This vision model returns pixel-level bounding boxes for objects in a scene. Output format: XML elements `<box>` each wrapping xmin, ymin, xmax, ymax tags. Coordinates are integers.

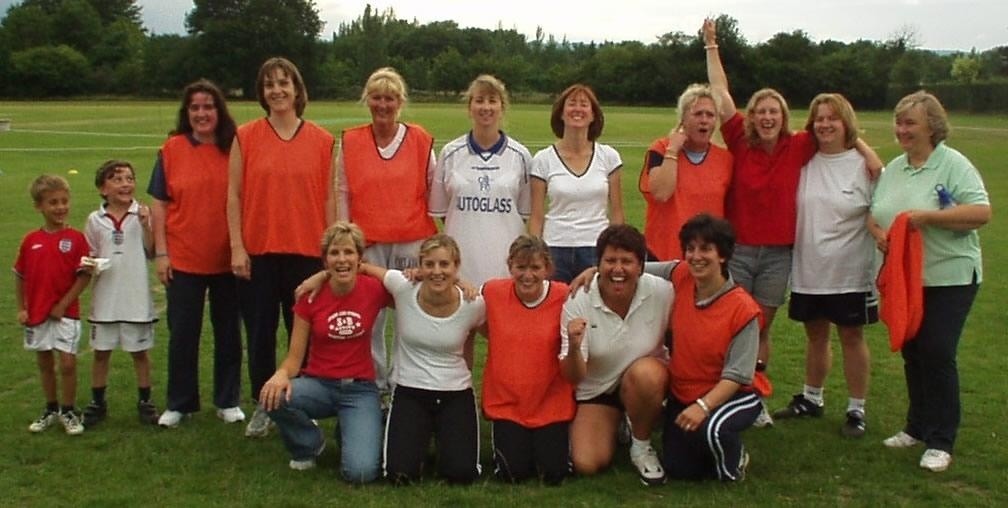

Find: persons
<box><xmin>637</xmin><ymin>85</ymin><xmax>734</xmax><ymax>265</ymax></box>
<box><xmin>13</xmin><ymin>174</ymin><xmax>90</xmax><ymax>441</ymax></box>
<box><xmin>557</xmin><ymin>222</ymin><xmax>677</xmax><ymax>486</ymax></box>
<box><xmin>865</xmin><ymin>90</ymin><xmax>992</xmax><ymax>469</ymax></box>
<box><xmin>565</xmin><ymin>211</ymin><xmax>766</xmax><ymax>488</ymax></box>
<box><xmin>770</xmin><ymin>92</ymin><xmax>887</xmax><ymax>439</ymax></box>
<box><xmin>291</xmin><ymin>233</ymin><xmax>488</xmax><ymax>480</ymax></box>
<box><xmin>144</xmin><ymin>79</ymin><xmax>249</xmax><ymax>429</ymax></box>
<box><xmin>224</xmin><ymin>56</ymin><xmax>337</xmax><ymax>438</ymax></box>
<box><xmin>529</xmin><ymin>83</ymin><xmax>625</xmax><ymax>279</ymax></box>
<box><xmin>323</xmin><ymin>67</ymin><xmax>441</xmax><ymax>419</ymax></box>
<box><xmin>82</xmin><ymin>158</ymin><xmax>157</xmax><ymax>429</ymax></box>
<box><xmin>703</xmin><ymin>15</ymin><xmax>880</xmax><ymax>434</ymax></box>
<box><xmin>398</xmin><ymin>233</ymin><xmax>574</xmax><ymax>485</ymax></box>
<box><xmin>427</xmin><ymin>75</ymin><xmax>538</xmax><ymax>378</ymax></box>
<box><xmin>258</xmin><ymin>221</ymin><xmax>396</xmax><ymax>487</ymax></box>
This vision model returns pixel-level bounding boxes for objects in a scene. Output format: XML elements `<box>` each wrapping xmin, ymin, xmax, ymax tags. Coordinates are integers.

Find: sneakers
<box><xmin>157</xmin><ymin>408</ymin><xmax>192</xmax><ymax>428</ymax></box>
<box><xmin>217</xmin><ymin>406</ymin><xmax>245</xmax><ymax>424</ymax></box>
<box><xmin>29</xmin><ymin>409</ymin><xmax>62</xmax><ymax>433</ymax></box>
<box><xmin>136</xmin><ymin>399</ymin><xmax>159</xmax><ymax>425</ymax></box>
<box><xmin>289</xmin><ymin>440</ymin><xmax>326</xmax><ymax>471</ymax></box>
<box><xmin>773</xmin><ymin>393</ymin><xmax>824</xmax><ymax>419</ymax></box>
<box><xmin>842</xmin><ymin>410</ymin><xmax>865</xmax><ymax>438</ymax></box>
<box><xmin>883</xmin><ymin>430</ymin><xmax>921</xmax><ymax>447</ymax></box>
<box><xmin>62</xmin><ymin>400</ymin><xmax>108</xmax><ymax>435</ymax></box>
<box><xmin>629</xmin><ymin>446</ymin><xmax>666</xmax><ymax>486</ymax></box>
<box><xmin>245</xmin><ymin>403</ymin><xmax>275</xmax><ymax>439</ymax></box>
<box><xmin>919</xmin><ymin>448</ymin><xmax>951</xmax><ymax>473</ymax></box>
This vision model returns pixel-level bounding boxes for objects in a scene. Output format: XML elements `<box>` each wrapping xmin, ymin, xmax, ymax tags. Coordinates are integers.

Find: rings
<box><xmin>685</xmin><ymin>424</ymin><xmax>690</xmax><ymax>430</ymax></box>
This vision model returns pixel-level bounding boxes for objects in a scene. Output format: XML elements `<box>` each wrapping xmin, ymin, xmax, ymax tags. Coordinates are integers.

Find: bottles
<box><xmin>935</xmin><ymin>181</ymin><xmax>973</xmax><ymax>240</ymax></box>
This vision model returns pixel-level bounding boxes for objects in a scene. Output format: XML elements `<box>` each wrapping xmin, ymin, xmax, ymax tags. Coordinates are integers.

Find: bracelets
<box><xmin>694</xmin><ymin>397</ymin><xmax>712</xmax><ymax>419</ymax></box>
<box><xmin>152</xmin><ymin>249</ymin><xmax>170</xmax><ymax>261</ymax></box>
<box><xmin>323</xmin><ymin>268</ymin><xmax>331</xmax><ymax>281</ymax></box>
<box><xmin>662</xmin><ymin>150</ymin><xmax>678</xmax><ymax>164</ymax></box>
<box><xmin>703</xmin><ymin>40</ymin><xmax>720</xmax><ymax>49</ymax></box>
